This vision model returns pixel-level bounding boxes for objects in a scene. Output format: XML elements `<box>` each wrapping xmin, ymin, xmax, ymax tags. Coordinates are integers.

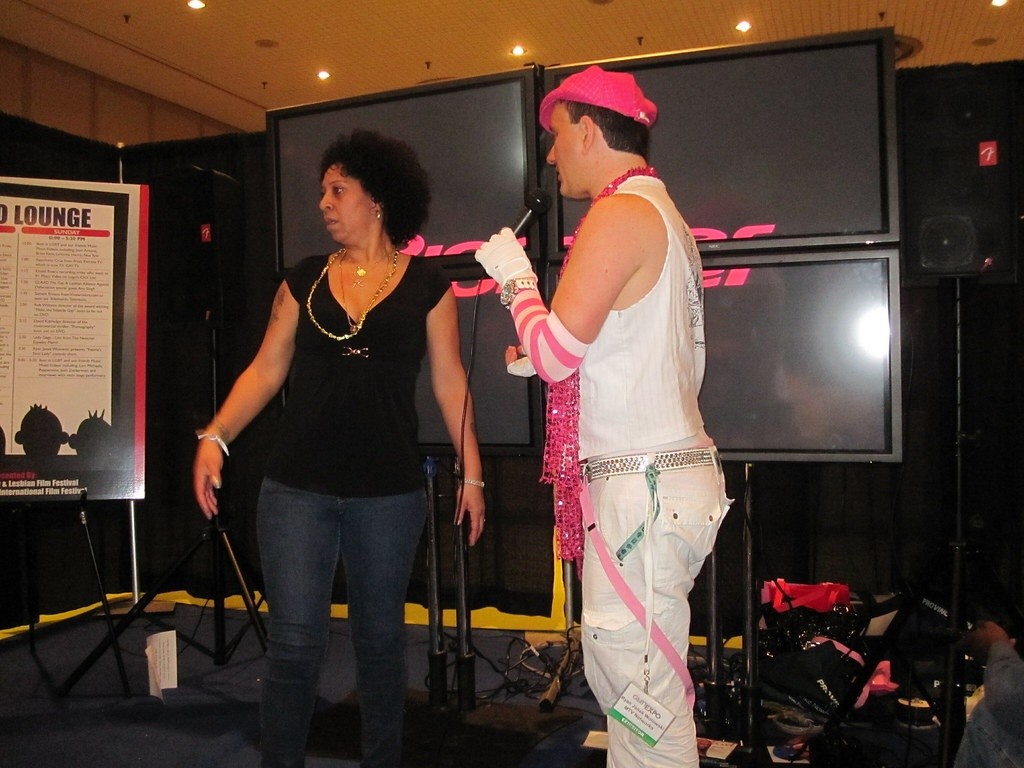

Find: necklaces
<box><xmin>307</xmin><ymin>245</ymin><xmax>399</xmax><ymax>341</ymax></box>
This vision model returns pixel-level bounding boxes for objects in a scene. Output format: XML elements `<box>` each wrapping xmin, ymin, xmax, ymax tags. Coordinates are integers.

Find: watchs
<box><xmin>500</xmin><ymin>278</ymin><xmax>537</xmax><ymax>306</ymax></box>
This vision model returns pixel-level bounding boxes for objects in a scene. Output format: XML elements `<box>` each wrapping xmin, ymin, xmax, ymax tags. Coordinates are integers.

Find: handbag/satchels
<box><xmin>751</xmin><ymin>577</ymin><xmax>961</xmax><ymax>752</ymax></box>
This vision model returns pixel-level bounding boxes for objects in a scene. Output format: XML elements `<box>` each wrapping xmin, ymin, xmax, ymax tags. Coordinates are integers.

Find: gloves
<box><xmin>475</xmin><ymin>225</ymin><xmax>538</xmax><ymax>286</ymax></box>
<box><xmin>506</xmin><ymin>345</ymin><xmax>537</xmax><ymax>377</ymax></box>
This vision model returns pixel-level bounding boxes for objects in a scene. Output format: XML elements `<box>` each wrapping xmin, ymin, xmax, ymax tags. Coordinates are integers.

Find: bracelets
<box><xmin>195</xmin><ymin>419</ymin><xmax>229</xmax><ymax>456</ymax></box>
<box><xmin>458</xmin><ymin>478</ymin><xmax>484</xmax><ymax>488</ymax></box>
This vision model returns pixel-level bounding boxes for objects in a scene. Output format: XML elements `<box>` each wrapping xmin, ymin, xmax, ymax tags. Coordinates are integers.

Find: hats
<box><xmin>539</xmin><ymin>64</ymin><xmax>657</xmax><ymax>134</ymax></box>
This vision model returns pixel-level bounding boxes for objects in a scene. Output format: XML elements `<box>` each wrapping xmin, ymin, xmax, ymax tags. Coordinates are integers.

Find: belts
<box><xmin>580</xmin><ymin>449</ymin><xmax>720</xmax><ymax>486</ymax></box>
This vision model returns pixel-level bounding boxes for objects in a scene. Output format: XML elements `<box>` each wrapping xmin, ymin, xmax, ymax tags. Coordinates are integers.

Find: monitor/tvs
<box><xmin>263</xmin><ymin>29</ymin><xmax>903</xmax><ymax>465</ymax></box>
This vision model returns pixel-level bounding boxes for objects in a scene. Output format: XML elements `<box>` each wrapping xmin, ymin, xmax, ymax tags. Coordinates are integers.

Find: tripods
<box><xmin>52</xmin><ymin>323</ymin><xmax>273</xmax><ymax>698</ymax></box>
<box><xmin>819</xmin><ymin>277</ymin><xmax>1024</xmax><ymax>768</ymax></box>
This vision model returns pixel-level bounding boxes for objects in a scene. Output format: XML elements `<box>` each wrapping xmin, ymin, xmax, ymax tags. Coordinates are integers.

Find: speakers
<box><xmin>149</xmin><ymin>136</ymin><xmax>247</xmax><ymax>331</ymax></box>
<box><xmin>894</xmin><ymin>62</ymin><xmax>1024</xmax><ymax>287</ymax></box>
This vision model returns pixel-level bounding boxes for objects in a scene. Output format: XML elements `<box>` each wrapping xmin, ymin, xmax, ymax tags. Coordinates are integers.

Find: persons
<box><xmin>952</xmin><ymin>620</ymin><xmax>1024</xmax><ymax>768</ymax></box>
<box><xmin>475</xmin><ymin>65</ymin><xmax>735</xmax><ymax>768</ymax></box>
<box><xmin>194</xmin><ymin>128</ymin><xmax>485</xmax><ymax>768</ymax></box>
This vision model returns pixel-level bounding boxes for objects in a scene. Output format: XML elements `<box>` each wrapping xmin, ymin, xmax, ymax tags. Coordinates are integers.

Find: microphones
<box><xmin>512</xmin><ymin>188</ymin><xmax>549</xmax><ymax>239</ymax></box>
